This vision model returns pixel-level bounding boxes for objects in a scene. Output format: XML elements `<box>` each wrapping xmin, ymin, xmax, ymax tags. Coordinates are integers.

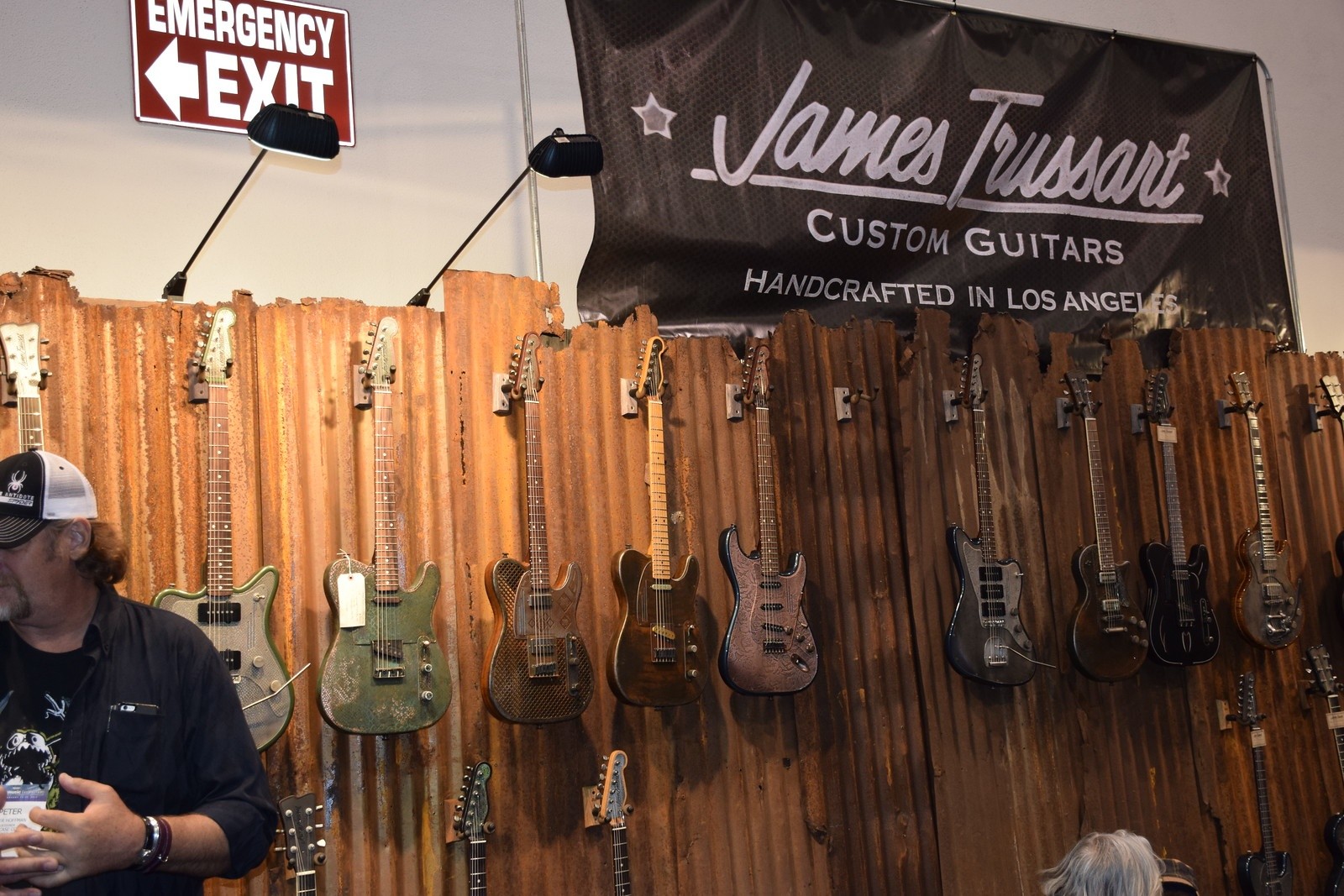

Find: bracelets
<box><xmin>142</xmin><ymin>816</ymin><xmax>172</xmax><ymax>874</ymax></box>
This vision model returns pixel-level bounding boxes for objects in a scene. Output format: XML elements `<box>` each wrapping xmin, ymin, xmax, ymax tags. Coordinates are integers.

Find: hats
<box><xmin>0</xmin><ymin>445</ymin><xmax>97</xmax><ymax>549</ymax></box>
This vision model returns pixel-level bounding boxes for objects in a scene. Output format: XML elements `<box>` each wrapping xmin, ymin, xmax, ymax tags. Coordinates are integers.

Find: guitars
<box><xmin>942</xmin><ymin>352</ymin><xmax>1057</xmax><ymax>687</ymax></box>
<box><xmin>1139</xmin><ymin>370</ymin><xmax>1221</xmax><ymax>667</ymax></box>
<box><xmin>1059</xmin><ymin>367</ymin><xmax>1150</xmax><ymax>685</ymax></box>
<box><xmin>0</xmin><ymin>320</ymin><xmax>54</xmax><ymax>453</ymax></box>
<box><xmin>1302</xmin><ymin>644</ymin><xmax>1344</xmax><ymax>896</ymax></box>
<box><xmin>717</xmin><ymin>343</ymin><xmax>822</xmax><ymax>698</ymax></box>
<box><xmin>273</xmin><ymin>791</ymin><xmax>327</xmax><ymax>896</ymax></box>
<box><xmin>1315</xmin><ymin>374</ymin><xmax>1343</xmax><ymax>575</ymax></box>
<box><xmin>607</xmin><ymin>335</ymin><xmax>710</xmax><ymax>708</ymax></box>
<box><xmin>452</xmin><ymin>761</ymin><xmax>497</xmax><ymax>895</ymax></box>
<box><xmin>479</xmin><ymin>331</ymin><xmax>595</xmax><ymax>726</ymax></box>
<box><xmin>314</xmin><ymin>315</ymin><xmax>454</xmax><ymax>737</ymax></box>
<box><xmin>149</xmin><ymin>307</ymin><xmax>296</xmax><ymax>753</ymax></box>
<box><xmin>591</xmin><ymin>749</ymin><xmax>635</xmax><ymax>896</ymax></box>
<box><xmin>1235</xmin><ymin>671</ymin><xmax>1294</xmax><ymax>896</ymax></box>
<box><xmin>1224</xmin><ymin>370</ymin><xmax>1306</xmax><ymax>652</ymax></box>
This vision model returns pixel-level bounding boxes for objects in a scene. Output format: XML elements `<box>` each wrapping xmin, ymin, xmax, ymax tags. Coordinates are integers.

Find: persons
<box><xmin>1038</xmin><ymin>830</ymin><xmax>1164</xmax><ymax>896</ymax></box>
<box><xmin>0</xmin><ymin>447</ymin><xmax>277</xmax><ymax>896</ymax></box>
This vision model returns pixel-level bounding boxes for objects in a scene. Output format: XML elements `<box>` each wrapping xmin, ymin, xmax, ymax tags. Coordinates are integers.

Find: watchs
<box><xmin>137</xmin><ymin>811</ymin><xmax>155</xmax><ymax>866</ymax></box>
